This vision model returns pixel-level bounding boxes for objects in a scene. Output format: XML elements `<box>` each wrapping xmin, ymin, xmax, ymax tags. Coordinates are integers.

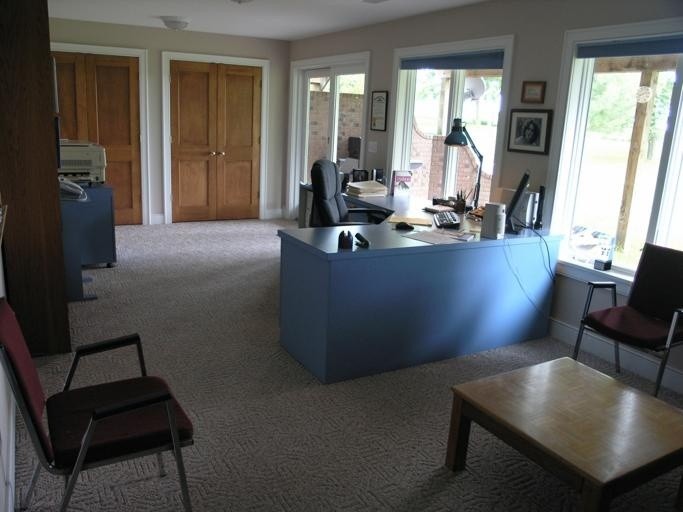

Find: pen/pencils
<box><xmin>456</xmin><ymin>189</ymin><xmax>473</xmax><ymax>200</ymax></box>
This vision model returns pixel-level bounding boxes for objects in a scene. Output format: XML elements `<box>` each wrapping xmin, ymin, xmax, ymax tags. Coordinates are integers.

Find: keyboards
<box><xmin>433</xmin><ymin>212</ymin><xmax>460</xmax><ymax>228</ymax></box>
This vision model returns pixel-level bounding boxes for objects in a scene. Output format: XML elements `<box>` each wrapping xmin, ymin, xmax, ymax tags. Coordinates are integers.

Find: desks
<box><xmin>277</xmin><ymin>185</ymin><xmax>561</xmax><ymax>382</ymax></box>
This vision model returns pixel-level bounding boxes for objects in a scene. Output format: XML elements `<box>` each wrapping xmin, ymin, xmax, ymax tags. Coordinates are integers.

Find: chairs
<box><xmin>308</xmin><ymin>160</ymin><xmax>387</xmax><ymax>227</ymax></box>
<box><xmin>573</xmin><ymin>242</ymin><xmax>683</xmax><ymax>398</ymax></box>
<box><xmin>1</xmin><ymin>296</ymin><xmax>194</xmax><ymax>512</ymax></box>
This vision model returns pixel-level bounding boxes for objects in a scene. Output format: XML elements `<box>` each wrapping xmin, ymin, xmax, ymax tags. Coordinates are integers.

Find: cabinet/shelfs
<box><xmin>0</xmin><ymin>0</ymin><xmax>72</xmax><ymax>358</ymax></box>
<box><xmin>61</xmin><ymin>185</ymin><xmax>117</xmax><ymax>301</ymax></box>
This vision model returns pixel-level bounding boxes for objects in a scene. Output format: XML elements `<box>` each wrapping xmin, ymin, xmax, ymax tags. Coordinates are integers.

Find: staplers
<box><xmin>355</xmin><ymin>233</ymin><xmax>369</xmax><ymax>249</ymax></box>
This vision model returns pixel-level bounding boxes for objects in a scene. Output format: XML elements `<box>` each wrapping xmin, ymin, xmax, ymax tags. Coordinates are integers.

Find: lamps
<box><xmin>444</xmin><ymin>118</ymin><xmax>484</xmax><ymax>210</ymax></box>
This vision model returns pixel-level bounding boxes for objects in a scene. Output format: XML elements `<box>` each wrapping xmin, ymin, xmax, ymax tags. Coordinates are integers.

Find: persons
<box><xmin>516</xmin><ymin>119</ymin><xmax>540</xmax><ymax>146</ymax></box>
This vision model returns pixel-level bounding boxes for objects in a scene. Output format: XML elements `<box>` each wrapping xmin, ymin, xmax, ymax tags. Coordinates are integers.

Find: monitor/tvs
<box><xmin>505</xmin><ymin>171</ymin><xmax>529</xmax><ymax>234</ymax></box>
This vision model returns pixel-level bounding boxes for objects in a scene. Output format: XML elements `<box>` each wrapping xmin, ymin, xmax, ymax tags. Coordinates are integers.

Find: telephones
<box><xmin>61</xmin><ymin>180</ymin><xmax>83</xmax><ymax>198</ymax></box>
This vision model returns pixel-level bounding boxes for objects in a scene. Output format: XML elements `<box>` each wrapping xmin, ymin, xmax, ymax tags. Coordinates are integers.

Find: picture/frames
<box><xmin>521</xmin><ymin>81</ymin><xmax>546</xmax><ymax>103</ymax></box>
<box><xmin>507</xmin><ymin>109</ymin><xmax>553</xmax><ymax>155</ymax></box>
<box><xmin>370</xmin><ymin>91</ymin><xmax>388</xmax><ymax>131</ymax></box>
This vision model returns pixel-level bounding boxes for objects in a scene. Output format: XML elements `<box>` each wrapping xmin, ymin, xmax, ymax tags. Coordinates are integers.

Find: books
<box><xmin>346</xmin><ymin>180</ymin><xmax>387</xmax><ymax>197</ymax></box>
<box><xmin>452</xmin><ymin>234</ymin><xmax>474</xmax><ymax>241</ymax></box>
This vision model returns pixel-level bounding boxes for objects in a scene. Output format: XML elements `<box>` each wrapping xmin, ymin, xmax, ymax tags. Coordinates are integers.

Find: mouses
<box><xmin>396</xmin><ymin>222</ymin><xmax>414</xmax><ymax>229</ymax></box>
